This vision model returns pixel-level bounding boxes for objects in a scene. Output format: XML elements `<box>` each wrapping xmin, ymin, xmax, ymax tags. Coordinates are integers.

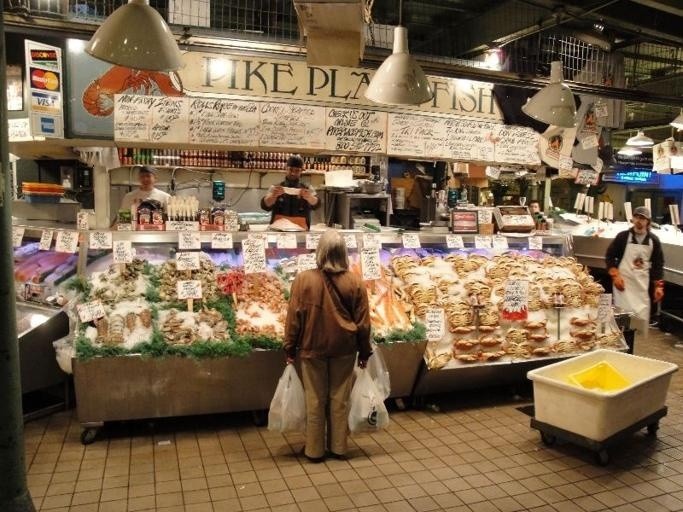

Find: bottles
<box><xmin>119</xmin><ymin>147</ymin><xmax>366</xmax><ymax>174</ymax></box>
<box><xmin>77</xmin><ymin>208</ymin><xmax>97</xmax><ymax>229</ymax></box>
<box><xmin>533</xmin><ymin>212</ymin><xmax>554</xmax><ymax>230</ymax></box>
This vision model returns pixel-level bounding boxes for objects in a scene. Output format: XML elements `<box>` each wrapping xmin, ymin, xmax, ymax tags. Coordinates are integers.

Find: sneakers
<box><xmin>301</xmin><ymin>445</ymin><xmax>345</xmax><ymax>462</ymax></box>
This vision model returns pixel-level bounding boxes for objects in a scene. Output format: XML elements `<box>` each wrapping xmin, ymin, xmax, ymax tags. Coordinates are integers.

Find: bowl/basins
<box><xmin>357</xmin><ymin>180</ymin><xmax>384</xmax><ymax>194</ymax></box>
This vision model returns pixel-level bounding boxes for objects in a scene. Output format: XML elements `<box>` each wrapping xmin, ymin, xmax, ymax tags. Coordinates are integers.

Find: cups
<box><xmin>520</xmin><ymin>197</ymin><xmax>527</xmax><ymax>206</ymax></box>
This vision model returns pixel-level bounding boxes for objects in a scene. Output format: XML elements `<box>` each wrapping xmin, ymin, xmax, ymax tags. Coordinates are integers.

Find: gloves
<box><xmin>607</xmin><ymin>267</ymin><xmax>625</xmax><ymax>292</ymax></box>
<box><xmin>653</xmin><ymin>280</ymin><xmax>664</xmax><ymax>302</ymax></box>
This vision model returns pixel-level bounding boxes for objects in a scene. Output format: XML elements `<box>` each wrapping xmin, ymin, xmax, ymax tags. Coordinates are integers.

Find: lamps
<box><xmin>616</xmin><ymin>106</ymin><xmax>683</xmax><ymax>156</ymax></box>
<box><xmin>364</xmin><ymin>2</ymin><xmax>435</xmax><ymax>105</ymax></box>
<box><xmin>521</xmin><ymin>1</ymin><xmax>576</xmax><ymax>130</ymax></box>
<box><xmin>83</xmin><ymin>2</ymin><xmax>184</xmax><ymax>73</ymax></box>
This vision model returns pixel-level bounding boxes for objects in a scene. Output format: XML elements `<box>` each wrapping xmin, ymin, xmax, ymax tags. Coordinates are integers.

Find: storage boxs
<box><xmin>526</xmin><ymin>343</ymin><xmax>681</xmax><ymax>441</ymax></box>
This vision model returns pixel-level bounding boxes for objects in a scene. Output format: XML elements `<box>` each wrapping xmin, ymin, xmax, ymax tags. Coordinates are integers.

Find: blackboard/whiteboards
<box><xmin>388</xmin><ymin>111</ymin><xmax>446</xmax><ymax>159</ymax></box>
<box><xmin>187</xmin><ymin>95</ymin><xmax>260</xmax><ymax>151</ymax></box>
<box><xmin>326</xmin><ymin>103</ymin><xmax>388</xmax><ymax>156</ymax></box>
<box><xmin>495</xmin><ymin>115</ymin><xmax>540</xmax><ymax>169</ymax></box>
<box><xmin>113</xmin><ymin>95</ymin><xmax>189</xmax><ymax>148</ymax></box>
<box><xmin>446</xmin><ymin>113</ymin><xmax>494</xmax><ymax>165</ymax></box>
<box><xmin>260</xmin><ymin>101</ymin><xmax>326</xmax><ymax>152</ymax></box>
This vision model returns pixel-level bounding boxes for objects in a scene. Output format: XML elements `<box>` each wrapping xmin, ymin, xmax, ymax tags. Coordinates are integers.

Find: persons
<box><xmin>283</xmin><ymin>229</ymin><xmax>373</xmax><ymax>463</ymax></box>
<box><xmin>112</xmin><ymin>164</ymin><xmax>171</xmax><ymax>230</ymax></box>
<box><xmin>602</xmin><ymin>206</ymin><xmax>665</xmax><ymax>354</ymax></box>
<box><xmin>260</xmin><ymin>155</ymin><xmax>321</xmax><ymax>231</ymax></box>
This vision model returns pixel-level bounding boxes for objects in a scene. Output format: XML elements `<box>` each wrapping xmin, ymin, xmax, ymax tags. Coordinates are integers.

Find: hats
<box><xmin>287</xmin><ymin>156</ymin><xmax>303</xmax><ymax>168</ymax></box>
<box><xmin>139</xmin><ymin>167</ymin><xmax>156</xmax><ymax>173</ymax></box>
<box><xmin>633</xmin><ymin>206</ymin><xmax>650</xmax><ymax>219</ymax></box>
